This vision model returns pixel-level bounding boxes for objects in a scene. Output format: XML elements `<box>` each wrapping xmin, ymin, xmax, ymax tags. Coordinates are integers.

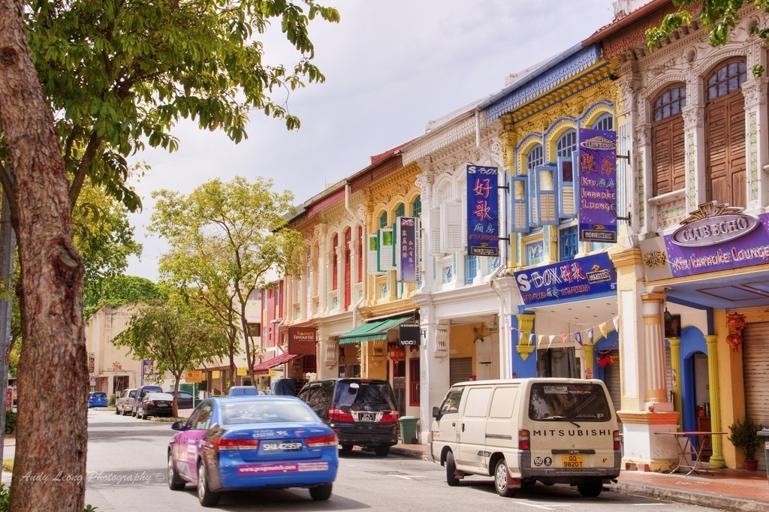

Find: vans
<box><xmin>298</xmin><ymin>378</ymin><xmax>401</xmax><ymax>459</ymax></box>
<box><xmin>430</xmin><ymin>376</ymin><xmax>623</xmax><ymax>497</ymax></box>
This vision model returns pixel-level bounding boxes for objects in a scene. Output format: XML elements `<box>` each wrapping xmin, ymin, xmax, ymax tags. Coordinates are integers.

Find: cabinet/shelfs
<box><xmin>699</xmin><ymin>415</ymin><xmax>713</xmax><ymax>456</ymax></box>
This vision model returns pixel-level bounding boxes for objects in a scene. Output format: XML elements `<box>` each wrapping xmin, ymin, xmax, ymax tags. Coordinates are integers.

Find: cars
<box><xmin>168</xmin><ymin>386</ymin><xmax>338</xmax><ymax>508</ymax></box>
<box><xmin>115</xmin><ymin>385</ymin><xmax>203</xmax><ymax>419</ymax></box>
<box><xmin>87</xmin><ymin>391</ymin><xmax>108</xmax><ymax>408</ymax></box>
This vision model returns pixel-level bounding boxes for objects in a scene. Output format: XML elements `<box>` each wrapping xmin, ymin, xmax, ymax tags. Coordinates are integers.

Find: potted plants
<box><xmin>727</xmin><ymin>417</ymin><xmax>763</xmax><ymax>470</ymax></box>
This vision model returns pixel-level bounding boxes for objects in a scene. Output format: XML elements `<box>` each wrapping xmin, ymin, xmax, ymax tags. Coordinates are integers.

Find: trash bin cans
<box><xmin>398</xmin><ymin>415</ymin><xmax>420</xmax><ymax>444</ymax></box>
<box><xmin>757</xmin><ymin>428</ymin><xmax>769</xmax><ymax>480</ymax></box>
<box><xmin>195</xmin><ymin>400</ymin><xmax>201</xmax><ymax>407</ymax></box>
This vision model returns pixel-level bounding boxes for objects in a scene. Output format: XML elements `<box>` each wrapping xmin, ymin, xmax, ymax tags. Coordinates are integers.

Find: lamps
<box><xmin>387</xmin><ymin>343</ymin><xmax>404</xmax><ymax>364</ymax></box>
<box><xmin>725</xmin><ymin>312</ymin><xmax>746</xmax><ymax>333</ymax></box>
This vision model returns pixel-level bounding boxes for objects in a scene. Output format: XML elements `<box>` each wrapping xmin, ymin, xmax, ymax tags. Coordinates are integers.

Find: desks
<box><xmin>654</xmin><ymin>430</ymin><xmax>728</xmax><ymax>476</ymax></box>
